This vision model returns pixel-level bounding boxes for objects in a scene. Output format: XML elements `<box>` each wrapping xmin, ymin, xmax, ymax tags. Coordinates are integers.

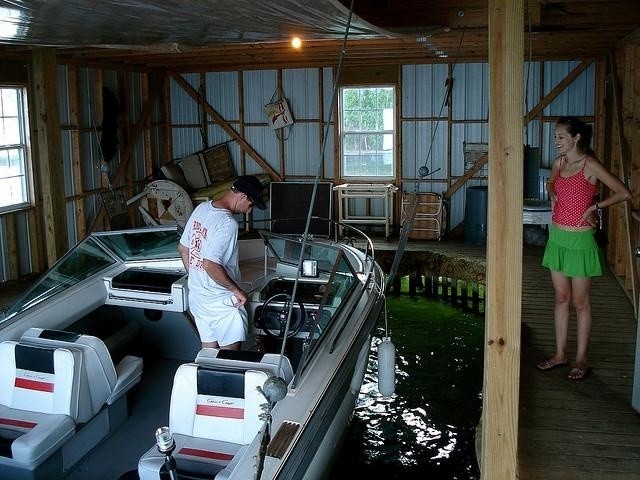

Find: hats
<box><xmin>231</xmin><ymin>175</ymin><xmax>267</xmax><ymax>210</ymax></box>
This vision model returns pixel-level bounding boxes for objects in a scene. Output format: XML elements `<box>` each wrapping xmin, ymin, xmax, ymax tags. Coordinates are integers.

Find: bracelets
<box><xmin>595</xmin><ymin>202</ymin><xmax>605</xmax><ymax>211</ymax></box>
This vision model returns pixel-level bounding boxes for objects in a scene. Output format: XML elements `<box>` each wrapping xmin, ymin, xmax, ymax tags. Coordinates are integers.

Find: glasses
<box><xmin>248</xmin><ymin>196</ymin><xmax>256</xmax><ymax>207</ymax></box>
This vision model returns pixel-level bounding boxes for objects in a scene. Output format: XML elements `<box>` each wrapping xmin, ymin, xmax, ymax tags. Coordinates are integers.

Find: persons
<box><xmin>536</xmin><ymin>118</ymin><xmax>632</xmax><ymax>380</ymax></box>
<box><xmin>177</xmin><ymin>175</ymin><xmax>267</xmax><ymax>351</ymax></box>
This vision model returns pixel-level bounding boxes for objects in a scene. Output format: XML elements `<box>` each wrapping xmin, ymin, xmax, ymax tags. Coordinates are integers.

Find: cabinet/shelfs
<box><xmin>332</xmin><ymin>183</ymin><xmax>392</xmax><ymax>243</ymax></box>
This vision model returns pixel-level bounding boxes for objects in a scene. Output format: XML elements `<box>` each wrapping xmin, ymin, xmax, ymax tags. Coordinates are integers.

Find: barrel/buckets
<box><xmin>465</xmin><ymin>186</ymin><xmax>486</xmax><ymax>242</ymax></box>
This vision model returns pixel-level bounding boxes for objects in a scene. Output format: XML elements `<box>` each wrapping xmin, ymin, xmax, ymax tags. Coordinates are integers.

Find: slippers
<box><xmin>536</xmin><ymin>358</ymin><xmax>568</xmax><ymax>371</ymax></box>
<box><xmin>567</xmin><ymin>367</ymin><xmax>590</xmax><ymax>381</ymax></box>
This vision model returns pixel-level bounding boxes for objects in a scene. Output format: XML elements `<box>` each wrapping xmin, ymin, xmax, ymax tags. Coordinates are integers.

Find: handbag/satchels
<box><xmin>265</xmin><ymin>87</ymin><xmax>294</xmax><ymax>141</ymax></box>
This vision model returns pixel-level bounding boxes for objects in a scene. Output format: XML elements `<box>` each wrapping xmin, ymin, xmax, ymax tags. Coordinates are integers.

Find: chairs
<box><xmin>136</xmin><ymin>347</ymin><xmax>295</xmax><ymax>480</ymax></box>
<box><xmin>0</xmin><ymin>329</ymin><xmax>145</xmax><ymax>472</ymax></box>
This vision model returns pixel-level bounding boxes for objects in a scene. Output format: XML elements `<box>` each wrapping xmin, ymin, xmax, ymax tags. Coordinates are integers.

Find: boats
<box><xmin>0</xmin><ymin>214</ymin><xmax>389</xmax><ymax>480</ymax></box>
